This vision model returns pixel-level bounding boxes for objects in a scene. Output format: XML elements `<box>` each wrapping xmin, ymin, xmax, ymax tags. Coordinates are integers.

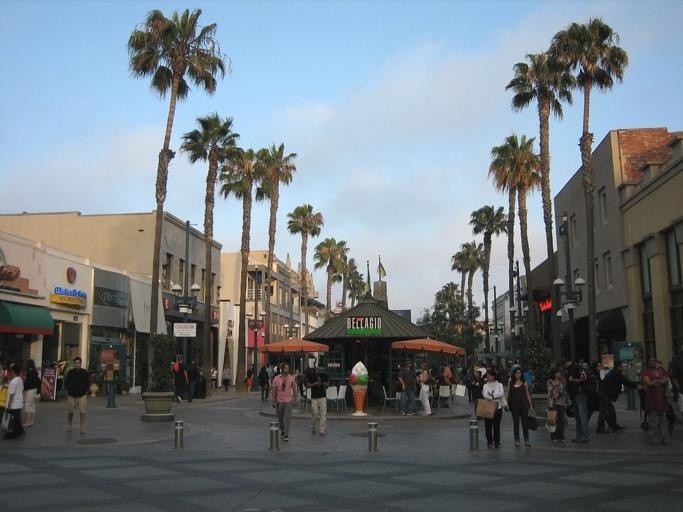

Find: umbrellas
<box><xmin>258</xmin><ymin>338</ymin><xmax>331</xmax><ymax>362</ymax></box>
<box><xmin>392</xmin><ymin>336</ymin><xmax>466</xmax><ymax>363</ymax></box>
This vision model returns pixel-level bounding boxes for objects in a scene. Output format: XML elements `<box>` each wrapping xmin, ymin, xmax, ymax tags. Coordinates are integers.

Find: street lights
<box><xmin>245</xmin><ymin>310</ymin><xmax>266</xmax><ymax>392</ymax></box>
<box><xmin>171</xmin><ymin>283</ymin><xmax>201</xmax><ymax>367</ymax></box>
<box><xmin>551</xmin><ymin>277</ymin><xmax>585</xmax><ymax>361</ymax></box>
<box><xmin>283</xmin><ymin>323</ymin><xmax>299</xmax><ymax>375</ymax></box>
<box><xmin>486</xmin><ymin>322</ymin><xmax>505</xmax><ymax>369</ymax></box>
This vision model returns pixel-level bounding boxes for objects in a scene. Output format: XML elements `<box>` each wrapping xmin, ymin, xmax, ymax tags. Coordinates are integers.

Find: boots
<box><xmin>80</xmin><ymin>413</ymin><xmax>87</xmax><ymax>434</ymax></box>
<box><xmin>67</xmin><ymin>413</ymin><xmax>73</xmax><ymax>432</ymax></box>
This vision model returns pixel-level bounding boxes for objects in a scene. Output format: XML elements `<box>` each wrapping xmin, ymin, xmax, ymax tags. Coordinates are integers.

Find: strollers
<box><xmin>637</xmin><ymin>388</ymin><xmax>682</xmax><ymax>434</ymax></box>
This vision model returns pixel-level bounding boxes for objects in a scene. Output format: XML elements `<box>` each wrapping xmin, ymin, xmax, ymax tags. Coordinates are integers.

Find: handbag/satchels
<box><xmin>1</xmin><ymin>413</ymin><xmax>14</xmax><ymax>433</ymax></box>
<box><xmin>565</xmin><ymin>381</ymin><xmax>581</xmax><ymax>394</ymax></box>
<box><xmin>665</xmin><ymin>377</ymin><xmax>674</xmax><ymax>398</ymax></box>
<box><xmin>476</xmin><ymin>398</ymin><xmax>576</xmax><ymax>433</ymax></box>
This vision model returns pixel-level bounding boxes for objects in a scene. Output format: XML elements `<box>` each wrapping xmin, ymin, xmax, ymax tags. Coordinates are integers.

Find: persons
<box><xmin>506</xmin><ymin>365</ymin><xmax>533</xmax><ymax>447</ymax></box>
<box><xmin>482</xmin><ymin>369</ymin><xmax>509</xmax><ymax>448</ymax></box>
<box><xmin>0</xmin><ymin>357</ymin><xmax>26</xmax><ymax>439</ymax></box>
<box><xmin>65</xmin><ymin>357</ymin><xmax>89</xmax><ymax>434</ymax></box>
<box><xmin>306</xmin><ymin>363</ymin><xmax>330</xmax><ymax>435</ymax></box>
<box><xmin>546</xmin><ymin>351</ymin><xmax>683</xmax><ymax>445</ymax></box>
<box><xmin>397</xmin><ymin>356</ymin><xmax>492</xmax><ymax>416</ymax></box>
<box><xmin>520</xmin><ymin>365</ymin><xmax>538</xmax><ymax>395</ymax></box>
<box><xmin>272</xmin><ymin>363</ymin><xmax>296</xmax><ymax>441</ymax></box>
<box><xmin>23</xmin><ymin>359</ymin><xmax>39</xmax><ymax>426</ymax></box>
<box><xmin>174</xmin><ymin>352</ymin><xmax>232</xmax><ymax>404</ymax></box>
<box><xmin>247</xmin><ymin>359</ymin><xmax>280</xmax><ymax>402</ymax></box>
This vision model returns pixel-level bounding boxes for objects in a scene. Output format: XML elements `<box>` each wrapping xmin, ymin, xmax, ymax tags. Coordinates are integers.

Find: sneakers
<box><xmin>281</xmin><ymin>425</ymin><xmax>326</xmax><ymax>441</ymax></box>
<box><xmin>399</xmin><ymin>409</ymin><xmax>431</xmax><ymax>417</ymax></box>
<box><xmin>487</xmin><ymin>426</ymin><xmax>671</xmax><ymax>448</ymax></box>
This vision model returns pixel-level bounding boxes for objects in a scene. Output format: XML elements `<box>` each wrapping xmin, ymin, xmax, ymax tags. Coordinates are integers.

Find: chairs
<box><xmin>437</xmin><ymin>385</ymin><xmax>451</xmax><ymax>409</ymax></box>
<box><xmin>326</xmin><ymin>386</ymin><xmax>338</xmax><ymax>411</ymax></box>
<box><xmin>382</xmin><ymin>385</ymin><xmax>399</xmax><ymax>414</ymax></box>
<box><xmin>300</xmin><ymin>387</ymin><xmax>311</xmax><ymax>410</ymax></box>
<box><xmin>338</xmin><ymin>385</ymin><xmax>348</xmax><ymax>412</ymax></box>
